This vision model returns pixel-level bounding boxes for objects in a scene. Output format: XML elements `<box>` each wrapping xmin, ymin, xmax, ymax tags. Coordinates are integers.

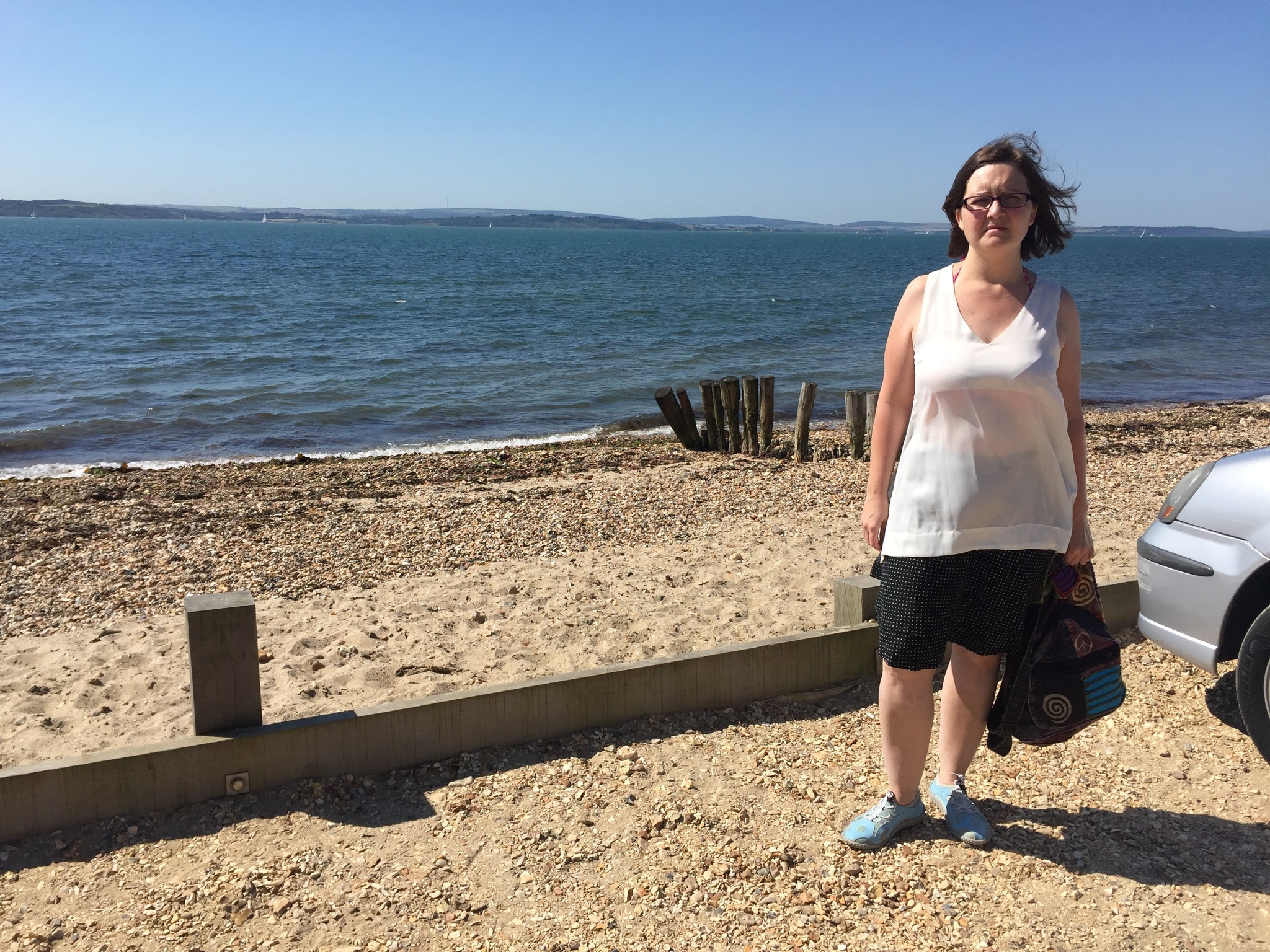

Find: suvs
<box><xmin>1132</xmin><ymin>445</ymin><xmax>1269</xmax><ymax>770</ymax></box>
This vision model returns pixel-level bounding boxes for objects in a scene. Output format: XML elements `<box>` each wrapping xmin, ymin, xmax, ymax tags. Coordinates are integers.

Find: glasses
<box><xmin>957</xmin><ymin>192</ymin><xmax>1033</xmax><ymax>211</ymax></box>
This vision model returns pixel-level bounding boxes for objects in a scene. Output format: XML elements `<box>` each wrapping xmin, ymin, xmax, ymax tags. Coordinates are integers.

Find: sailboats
<box><xmin>183</xmin><ymin>214</ymin><xmax>187</xmax><ymax>221</ymax></box>
<box><xmin>261</xmin><ymin>214</ymin><xmax>267</xmax><ymax>223</ymax></box>
<box><xmin>489</xmin><ymin>221</ymin><xmax>492</xmax><ymax>228</ymax></box>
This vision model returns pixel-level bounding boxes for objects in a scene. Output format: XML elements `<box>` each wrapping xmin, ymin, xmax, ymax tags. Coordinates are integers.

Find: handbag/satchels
<box><xmin>984</xmin><ymin>547</ymin><xmax>1126</xmax><ymax>757</ymax></box>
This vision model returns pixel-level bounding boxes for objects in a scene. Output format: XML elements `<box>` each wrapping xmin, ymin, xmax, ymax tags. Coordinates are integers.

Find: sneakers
<box><xmin>841</xmin><ymin>787</ymin><xmax>925</xmax><ymax>849</ymax></box>
<box><xmin>927</xmin><ymin>768</ymin><xmax>992</xmax><ymax>844</ymax></box>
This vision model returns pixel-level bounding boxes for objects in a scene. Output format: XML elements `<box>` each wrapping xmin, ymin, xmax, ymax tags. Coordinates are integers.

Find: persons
<box><xmin>839</xmin><ymin>133</ymin><xmax>1095</xmax><ymax>849</ymax></box>
